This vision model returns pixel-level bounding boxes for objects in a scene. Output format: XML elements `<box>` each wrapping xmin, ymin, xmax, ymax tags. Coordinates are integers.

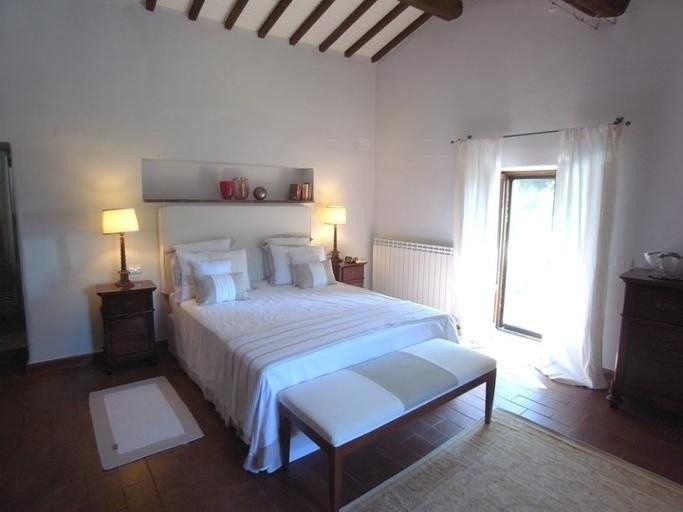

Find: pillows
<box><xmin>191</xmin><ymin>272</ymin><xmax>249</xmax><ymax>306</ymax></box>
<box><xmin>294</xmin><ymin>259</ymin><xmax>338</xmax><ymax>290</ymax></box>
<box><xmin>172</xmin><ymin>237</ymin><xmax>232</xmax><ymax>304</ymax></box>
<box><xmin>266</xmin><ymin>245</ymin><xmax>322</xmax><ymax>286</ymax></box>
<box><xmin>261</xmin><ymin>237</ymin><xmax>310</xmax><ymax>280</ymax></box>
<box><xmin>174</xmin><ymin>248</ymin><xmax>253</xmax><ymax>300</ymax></box>
<box><xmin>287</xmin><ymin>251</ymin><xmax>320</xmax><ymax>285</ymax></box>
<box><xmin>187</xmin><ymin>259</ymin><xmax>232</xmax><ymax>298</ymax></box>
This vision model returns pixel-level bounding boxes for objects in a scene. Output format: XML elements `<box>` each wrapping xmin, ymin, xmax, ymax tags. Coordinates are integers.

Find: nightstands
<box><xmin>332</xmin><ymin>258</ymin><xmax>368</xmax><ymax>288</ymax></box>
<box><xmin>96</xmin><ymin>279</ymin><xmax>157</xmax><ymax>376</ymax></box>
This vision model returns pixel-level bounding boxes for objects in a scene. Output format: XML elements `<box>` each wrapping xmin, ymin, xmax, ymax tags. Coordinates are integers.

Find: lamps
<box><xmin>322</xmin><ymin>207</ymin><xmax>347</xmax><ymax>263</ymax></box>
<box><xmin>100</xmin><ymin>208</ymin><xmax>140</xmax><ymax>288</ymax></box>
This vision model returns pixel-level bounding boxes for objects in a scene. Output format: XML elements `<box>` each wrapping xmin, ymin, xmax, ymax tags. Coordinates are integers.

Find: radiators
<box><xmin>372</xmin><ymin>236</ymin><xmax>455</xmax><ymax>313</ymax></box>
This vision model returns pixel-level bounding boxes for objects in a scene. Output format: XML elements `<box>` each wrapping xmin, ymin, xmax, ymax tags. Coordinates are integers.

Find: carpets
<box><xmin>337</xmin><ymin>405</ymin><xmax>683</xmax><ymax>511</ymax></box>
<box><xmin>88</xmin><ymin>375</ymin><xmax>205</xmax><ymax>471</ymax></box>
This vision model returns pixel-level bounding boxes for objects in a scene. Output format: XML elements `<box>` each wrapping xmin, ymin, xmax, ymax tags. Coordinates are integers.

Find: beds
<box><xmin>157</xmin><ymin>204</ymin><xmax>462</xmax><ymax>475</ymax></box>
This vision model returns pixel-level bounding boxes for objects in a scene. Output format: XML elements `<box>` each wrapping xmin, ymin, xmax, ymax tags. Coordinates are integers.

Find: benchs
<box><xmin>276</xmin><ymin>337</ymin><xmax>497</xmax><ymax>512</ymax></box>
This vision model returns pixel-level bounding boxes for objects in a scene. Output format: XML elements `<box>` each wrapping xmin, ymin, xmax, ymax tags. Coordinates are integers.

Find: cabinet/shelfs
<box><xmin>606</xmin><ymin>266</ymin><xmax>682</xmax><ymax>418</ymax></box>
